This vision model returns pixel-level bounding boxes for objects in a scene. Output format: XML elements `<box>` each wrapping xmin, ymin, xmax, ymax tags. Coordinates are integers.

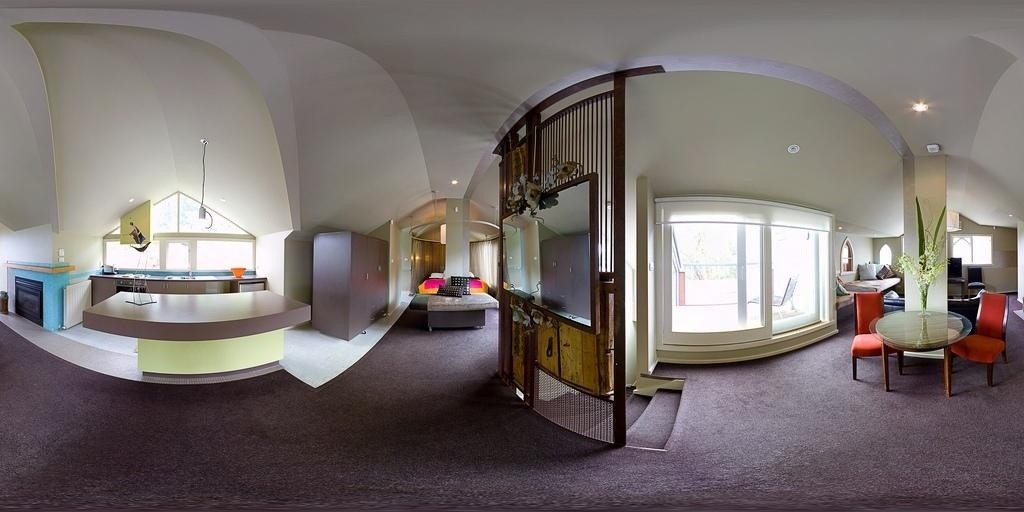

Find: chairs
<box><xmin>746</xmin><ymin>276</ymin><xmax>798</xmax><ymax>319</ymax></box>
<box><xmin>967</xmin><ymin>267</ymin><xmax>986</xmax><ymax>299</ymax></box>
<box><xmin>850</xmin><ymin>291</ymin><xmax>904</xmax><ymax>380</ymax></box>
<box><xmin>948</xmin><ymin>292</ymin><xmax>1009</xmax><ymax>388</ymax></box>
<box><xmin>948</xmin><ymin>258</ymin><xmax>965</xmax><ymax>299</ymax></box>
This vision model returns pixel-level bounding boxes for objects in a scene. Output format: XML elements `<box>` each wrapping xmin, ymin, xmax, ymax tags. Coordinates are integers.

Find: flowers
<box><xmin>893</xmin><ymin>203</ymin><xmax>953</xmax><ymax>283</ymax></box>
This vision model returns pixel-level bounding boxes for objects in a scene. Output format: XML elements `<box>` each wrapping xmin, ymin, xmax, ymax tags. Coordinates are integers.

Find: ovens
<box><xmin>15</xmin><ymin>277</ymin><xmax>43</xmax><ymax>328</ymax></box>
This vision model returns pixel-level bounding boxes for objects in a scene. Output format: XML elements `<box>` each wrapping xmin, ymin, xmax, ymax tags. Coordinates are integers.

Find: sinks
<box><xmin>180</xmin><ymin>276</ymin><xmax>195</xmax><ymax>280</ymax></box>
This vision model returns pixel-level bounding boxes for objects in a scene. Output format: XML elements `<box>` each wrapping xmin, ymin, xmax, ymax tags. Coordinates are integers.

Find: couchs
<box><xmin>882</xmin><ymin>289</ymin><xmax>987</xmax><ymax>335</ymax></box>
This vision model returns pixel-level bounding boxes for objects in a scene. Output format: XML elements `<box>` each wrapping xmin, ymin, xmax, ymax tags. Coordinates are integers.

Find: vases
<box><xmin>916</xmin><ymin>317</ymin><xmax>930</xmax><ymax>349</ymax></box>
<box><xmin>914</xmin><ymin>279</ymin><xmax>933</xmax><ymax>316</ymax></box>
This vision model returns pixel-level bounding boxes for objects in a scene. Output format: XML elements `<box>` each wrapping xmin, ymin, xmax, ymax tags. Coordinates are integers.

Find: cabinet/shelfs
<box><xmin>312</xmin><ymin>231</ymin><xmax>389</xmax><ymax>341</ymax></box>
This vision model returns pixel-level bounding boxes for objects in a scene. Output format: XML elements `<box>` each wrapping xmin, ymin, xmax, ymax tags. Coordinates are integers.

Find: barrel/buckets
<box><xmin>0</xmin><ymin>298</ymin><xmax>8</xmax><ymax>314</ymax></box>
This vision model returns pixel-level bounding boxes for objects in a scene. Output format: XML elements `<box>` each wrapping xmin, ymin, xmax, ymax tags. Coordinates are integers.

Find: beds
<box><xmin>417</xmin><ymin>273</ymin><xmax>489</xmax><ymax>294</ymax></box>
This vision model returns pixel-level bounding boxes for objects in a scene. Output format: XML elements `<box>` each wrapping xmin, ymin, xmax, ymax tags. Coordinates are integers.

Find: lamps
<box><xmin>198</xmin><ymin>139</ymin><xmax>213</xmax><ymax>231</ymax></box>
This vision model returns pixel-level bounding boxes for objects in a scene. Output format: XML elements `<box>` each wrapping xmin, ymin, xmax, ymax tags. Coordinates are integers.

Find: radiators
<box><xmin>62</xmin><ymin>280</ymin><xmax>91</xmax><ymax>330</ymax></box>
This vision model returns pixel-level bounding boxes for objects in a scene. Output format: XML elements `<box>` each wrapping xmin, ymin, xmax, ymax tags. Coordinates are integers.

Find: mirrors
<box><xmin>500</xmin><ymin>172</ymin><xmax>601</xmax><ymax>336</ymax></box>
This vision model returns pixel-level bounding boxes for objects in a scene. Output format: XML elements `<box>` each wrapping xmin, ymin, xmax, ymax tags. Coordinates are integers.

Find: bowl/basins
<box><xmin>231</xmin><ymin>268</ymin><xmax>246</xmax><ymax>278</ymax></box>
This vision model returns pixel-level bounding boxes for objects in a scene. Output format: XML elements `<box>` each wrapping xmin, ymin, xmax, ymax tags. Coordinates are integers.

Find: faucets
<box><xmin>188</xmin><ymin>271</ymin><xmax>193</xmax><ymax>277</ymax></box>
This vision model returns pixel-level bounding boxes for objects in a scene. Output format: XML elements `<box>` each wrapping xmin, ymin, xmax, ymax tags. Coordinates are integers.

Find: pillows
<box><xmin>450</xmin><ymin>276</ymin><xmax>471</xmax><ymax>295</ymax></box>
<box><xmin>469</xmin><ymin>272</ymin><xmax>474</xmax><ymax>277</ymax></box>
<box><xmin>429</xmin><ymin>273</ymin><xmax>444</xmax><ymax>279</ymax></box>
<box><xmin>437</xmin><ymin>285</ymin><xmax>464</xmax><ymax>297</ymax></box>
<box><xmin>858</xmin><ymin>263</ymin><xmax>896</xmax><ymax>280</ymax></box>
<box><xmin>836</xmin><ymin>279</ymin><xmax>850</xmax><ymax>296</ymax></box>
<box><xmin>837</xmin><ymin>276</ymin><xmax>849</xmax><ymax>289</ymax></box>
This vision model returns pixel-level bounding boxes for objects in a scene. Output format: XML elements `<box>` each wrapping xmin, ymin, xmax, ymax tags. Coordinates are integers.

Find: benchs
<box><xmin>410</xmin><ymin>293</ymin><xmax>500</xmax><ymax>332</ymax></box>
<box><xmin>847</xmin><ymin>277</ymin><xmax>902</xmax><ymax>309</ymax></box>
<box><xmin>836</xmin><ymin>283</ymin><xmax>876</xmax><ymax>329</ymax></box>
